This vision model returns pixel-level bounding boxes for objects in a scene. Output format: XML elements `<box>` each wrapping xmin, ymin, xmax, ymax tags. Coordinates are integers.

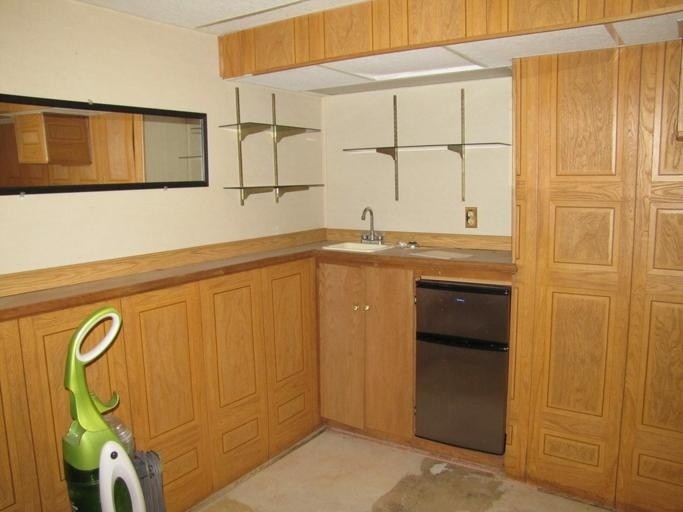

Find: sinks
<box><xmin>322</xmin><ymin>241</ymin><xmax>396</xmax><ymax>252</ymax></box>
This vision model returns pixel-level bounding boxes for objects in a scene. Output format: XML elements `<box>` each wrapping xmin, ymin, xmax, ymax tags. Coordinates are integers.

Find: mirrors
<box><xmin>0</xmin><ymin>93</ymin><xmax>209</xmax><ymax>196</ymax></box>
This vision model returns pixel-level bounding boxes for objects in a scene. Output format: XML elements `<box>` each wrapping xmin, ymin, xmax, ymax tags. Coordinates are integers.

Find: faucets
<box><xmin>360</xmin><ymin>206</ymin><xmax>375</xmax><ymax>241</ymax></box>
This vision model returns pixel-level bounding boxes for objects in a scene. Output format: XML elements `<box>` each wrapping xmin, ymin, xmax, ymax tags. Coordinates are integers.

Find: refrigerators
<box><xmin>412</xmin><ymin>277</ymin><xmax>513</xmax><ymax>457</ymax></box>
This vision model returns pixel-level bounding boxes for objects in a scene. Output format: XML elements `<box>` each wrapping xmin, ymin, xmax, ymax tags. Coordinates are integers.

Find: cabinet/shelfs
<box><xmin>0</xmin><ymin>102</ymin><xmax>145</xmax><ymax>187</ymax></box>
<box><xmin>1</xmin><ymin>254</ymin><xmax>319</xmax><ymax>510</ymax></box>
<box><xmin>320</xmin><ymin>248</ymin><xmax>415</xmax><ymax>447</ymax></box>
<box><xmin>219</xmin><ymin>122</ymin><xmax>325</xmax><ymax>205</ymax></box>
<box><xmin>502</xmin><ymin>39</ymin><xmax>683</xmax><ymax>512</ymax></box>
